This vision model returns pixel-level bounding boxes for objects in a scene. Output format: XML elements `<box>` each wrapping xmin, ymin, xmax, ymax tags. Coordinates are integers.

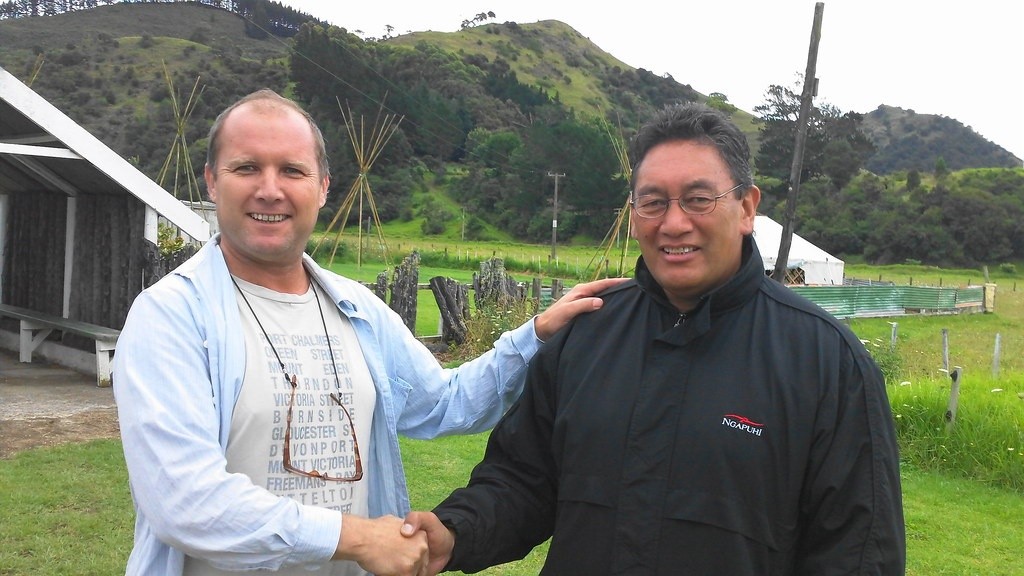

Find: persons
<box><xmin>109</xmin><ymin>87</ymin><xmax>632</xmax><ymax>576</ymax></box>
<box><xmin>401</xmin><ymin>104</ymin><xmax>906</xmax><ymax>576</ymax></box>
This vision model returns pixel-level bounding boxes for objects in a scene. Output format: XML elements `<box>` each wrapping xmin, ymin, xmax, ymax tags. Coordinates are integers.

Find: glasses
<box><xmin>283</xmin><ymin>374</ymin><xmax>367</xmax><ymax>482</ymax></box>
<box><xmin>631</xmin><ymin>178</ymin><xmax>744</xmax><ymax>219</ymax></box>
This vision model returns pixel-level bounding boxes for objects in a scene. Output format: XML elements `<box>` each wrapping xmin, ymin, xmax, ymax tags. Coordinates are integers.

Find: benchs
<box><xmin>1</xmin><ymin>303</ymin><xmax>122</xmax><ymax>388</ymax></box>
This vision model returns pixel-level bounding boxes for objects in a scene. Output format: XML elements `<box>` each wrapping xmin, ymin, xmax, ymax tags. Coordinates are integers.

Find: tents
<box><xmin>751</xmin><ymin>215</ymin><xmax>845</xmax><ymax>286</ymax></box>
